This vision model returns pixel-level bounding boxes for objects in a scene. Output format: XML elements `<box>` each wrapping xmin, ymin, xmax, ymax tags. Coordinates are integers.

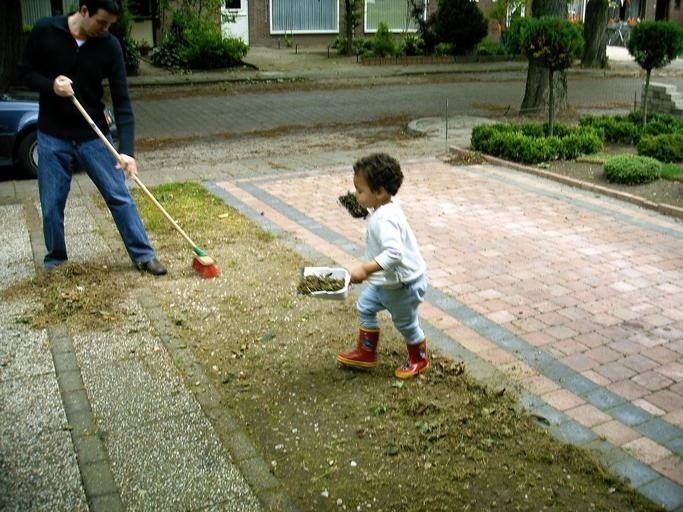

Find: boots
<box><xmin>395</xmin><ymin>339</ymin><xmax>433</xmax><ymax>380</ymax></box>
<box><xmin>335</xmin><ymin>328</ymin><xmax>381</xmax><ymax>368</ymax></box>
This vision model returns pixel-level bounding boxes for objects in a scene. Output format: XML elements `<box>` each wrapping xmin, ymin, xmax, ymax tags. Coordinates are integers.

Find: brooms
<box><xmin>68</xmin><ymin>95</ymin><xmax>219</xmax><ymax>278</ymax></box>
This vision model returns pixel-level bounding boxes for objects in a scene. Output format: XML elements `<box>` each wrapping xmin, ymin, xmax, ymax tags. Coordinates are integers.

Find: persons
<box><xmin>19</xmin><ymin>0</ymin><xmax>168</xmax><ymax>276</ymax></box>
<box><xmin>336</xmin><ymin>152</ymin><xmax>431</xmax><ymax>379</ymax></box>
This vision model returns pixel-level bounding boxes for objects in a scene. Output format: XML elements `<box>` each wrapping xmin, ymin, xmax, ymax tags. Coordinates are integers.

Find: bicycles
<box><xmin>608</xmin><ymin>17</ymin><xmax>631</xmax><ymax>47</ymax></box>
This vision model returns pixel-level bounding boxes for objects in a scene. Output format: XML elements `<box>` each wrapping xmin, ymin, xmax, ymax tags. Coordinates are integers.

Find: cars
<box><xmin>0</xmin><ymin>87</ymin><xmax>119</xmax><ymax>179</ymax></box>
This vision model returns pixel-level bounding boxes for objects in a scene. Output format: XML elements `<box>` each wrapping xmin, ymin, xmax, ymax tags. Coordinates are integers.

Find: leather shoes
<box><xmin>41</xmin><ymin>251</ymin><xmax>70</xmax><ymax>266</ymax></box>
<box><xmin>139</xmin><ymin>255</ymin><xmax>167</xmax><ymax>277</ymax></box>
